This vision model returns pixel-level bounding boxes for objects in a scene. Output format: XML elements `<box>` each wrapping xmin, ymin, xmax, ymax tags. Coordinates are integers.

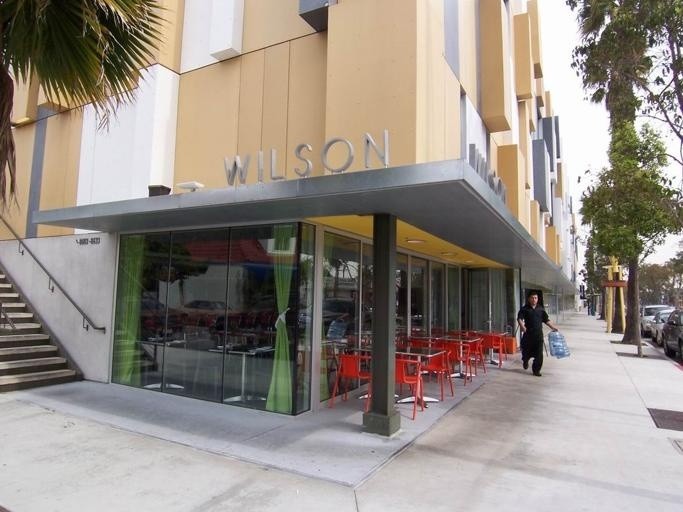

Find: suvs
<box><xmin>640</xmin><ymin>305</ymin><xmax>670</xmax><ymax>337</ymax></box>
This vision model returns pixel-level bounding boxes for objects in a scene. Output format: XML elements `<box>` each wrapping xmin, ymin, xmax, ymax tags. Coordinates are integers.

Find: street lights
<box><xmin>601</xmin><ymin>256</ymin><xmax>626</xmax><ymax>334</ymax></box>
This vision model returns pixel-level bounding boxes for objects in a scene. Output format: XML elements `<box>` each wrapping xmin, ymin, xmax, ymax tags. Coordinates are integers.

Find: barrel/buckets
<box><xmin>327</xmin><ymin>318</ymin><xmax>348</xmax><ymax>339</ymax></box>
<box><xmin>549</xmin><ymin>329</ymin><xmax>570</xmax><ymax>358</ymax></box>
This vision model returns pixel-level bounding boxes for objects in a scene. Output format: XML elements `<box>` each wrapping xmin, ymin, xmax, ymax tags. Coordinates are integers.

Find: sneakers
<box><xmin>533</xmin><ymin>369</ymin><xmax>541</xmax><ymax>376</ymax></box>
<box><xmin>523</xmin><ymin>361</ymin><xmax>528</xmax><ymax>369</ymax></box>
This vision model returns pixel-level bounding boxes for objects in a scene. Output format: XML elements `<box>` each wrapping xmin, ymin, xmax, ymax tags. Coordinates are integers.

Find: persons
<box><xmin>336</xmin><ymin>291</ymin><xmax>354</xmax><ymax>333</ymax></box>
<box><xmin>517</xmin><ymin>292</ymin><xmax>559</xmax><ymax>376</ymax></box>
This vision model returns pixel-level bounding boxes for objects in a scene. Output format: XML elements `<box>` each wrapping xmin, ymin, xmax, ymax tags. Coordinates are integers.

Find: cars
<box><xmin>297</xmin><ymin>297</ymin><xmax>372</xmax><ymax>342</ymax></box>
<box><xmin>139</xmin><ymin>298</ymin><xmax>187</xmax><ymax>332</ymax></box>
<box><xmin>177</xmin><ymin>299</ymin><xmax>239</xmax><ymax>326</ymax></box>
<box><xmin>649</xmin><ymin>309</ymin><xmax>673</xmax><ymax>345</ymax></box>
<box><xmin>660</xmin><ymin>310</ymin><xmax>683</xmax><ymax>361</ymax></box>
<box><xmin>214</xmin><ymin>295</ymin><xmax>295</xmax><ymax>327</ymax></box>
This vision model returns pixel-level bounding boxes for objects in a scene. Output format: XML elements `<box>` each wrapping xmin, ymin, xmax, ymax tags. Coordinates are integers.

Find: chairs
<box><xmin>325</xmin><ymin>327</ymin><xmax>507</xmax><ymax>420</ymax></box>
<box><xmin>138</xmin><ymin>306</ymin><xmax>327</xmax><ymax>407</ymax></box>
